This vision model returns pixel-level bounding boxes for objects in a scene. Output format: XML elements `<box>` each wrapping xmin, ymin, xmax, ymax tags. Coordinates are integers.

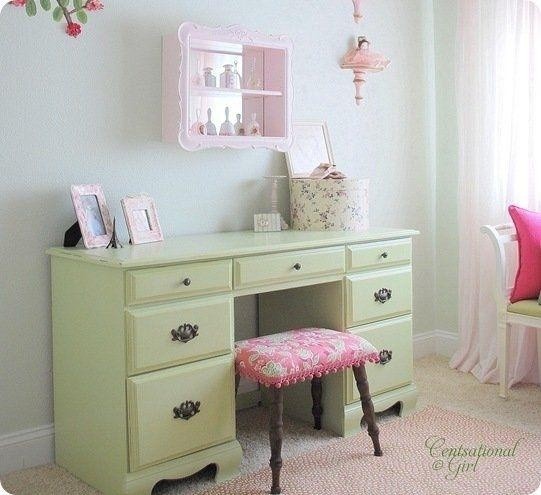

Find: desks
<box><xmin>46</xmin><ymin>227</ymin><xmax>422</xmax><ymax>495</ymax></box>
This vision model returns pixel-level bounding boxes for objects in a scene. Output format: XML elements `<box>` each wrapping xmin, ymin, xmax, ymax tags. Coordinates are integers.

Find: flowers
<box><xmin>11</xmin><ymin>0</ymin><xmax>106</xmax><ymax>38</ymax></box>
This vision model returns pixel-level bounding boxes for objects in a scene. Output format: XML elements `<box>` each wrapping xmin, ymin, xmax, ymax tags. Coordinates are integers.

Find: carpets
<box><xmin>203</xmin><ymin>404</ymin><xmax>540</xmax><ymax>494</ymax></box>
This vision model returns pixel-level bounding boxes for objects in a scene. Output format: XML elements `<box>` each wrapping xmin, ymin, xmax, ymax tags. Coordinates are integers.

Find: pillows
<box><xmin>507</xmin><ymin>204</ymin><xmax>540</xmax><ymax>305</ymax></box>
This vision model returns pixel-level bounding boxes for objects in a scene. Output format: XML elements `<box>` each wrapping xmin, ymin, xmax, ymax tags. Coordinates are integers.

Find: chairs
<box><xmin>481</xmin><ymin>223</ymin><xmax>540</xmax><ymax>397</ymax></box>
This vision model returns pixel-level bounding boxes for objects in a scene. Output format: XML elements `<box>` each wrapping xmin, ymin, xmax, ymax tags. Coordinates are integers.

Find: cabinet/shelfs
<box><xmin>162</xmin><ymin>21</ymin><xmax>295</xmax><ymax>154</ymax></box>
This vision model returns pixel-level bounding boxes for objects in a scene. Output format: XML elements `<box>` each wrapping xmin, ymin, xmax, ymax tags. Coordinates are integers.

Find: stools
<box><xmin>232</xmin><ymin>325</ymin><xmax>384</xmax><ymax>495</ymax></box>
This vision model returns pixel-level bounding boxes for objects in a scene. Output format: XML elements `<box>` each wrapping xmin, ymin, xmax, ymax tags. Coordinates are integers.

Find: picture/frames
<box><xmin>283</xmin><ymin>118</ymin><xmax>335</xmax><ymax>177</ymax></box>
<box><xmin>70</xmin><ymin>182</ymin><xmax>112</xmax><ymax>249</ymax></box>
<box><xmin>119</xmin><ymin>196</ymin><xmax>163</xmax><ymax>246</ymax></box>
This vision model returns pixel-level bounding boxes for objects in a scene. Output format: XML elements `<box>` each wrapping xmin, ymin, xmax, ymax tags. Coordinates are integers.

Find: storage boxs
<box><xmin>290</xmin><ymin>177</ymin><xmax>373</xmax><ymax>233</ymax></box>
